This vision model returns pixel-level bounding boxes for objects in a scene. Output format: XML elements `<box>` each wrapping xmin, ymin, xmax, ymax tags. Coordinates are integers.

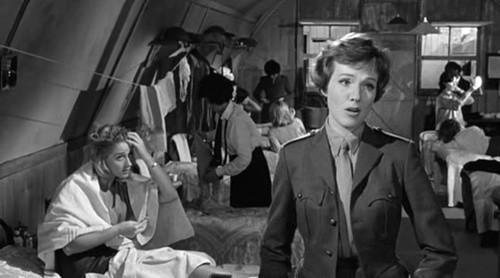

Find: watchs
<box><xmin>150</xmin><ymin>161</ymin><xmax>160</xmax><ymax>170</ymax></box>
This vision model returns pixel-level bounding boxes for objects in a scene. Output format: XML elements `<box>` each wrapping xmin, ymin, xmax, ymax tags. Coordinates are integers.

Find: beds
<box><xmin>0</xmin><ymin>244</ymin><xmax>285</xmax><ymax>278</ymax></box>
<box><xmin>459</xmin><ymin>160</ymin><xmax>500</xmax><ymax>242</ymax></box>
<box><xmin>418</xmin><ymin>127</ymin><xmax>448</xmax><ymax>195</ymax></box>
<box><xmin>435</xmin><ymin>144</ymin><xmax>500</xmax><ymax>205</ymax></box>
<box><xmin>261</xmin><ymin>135</ymin><xmax>270</xmax><ymax>149</ymax></box>
<box><xmin>167</xmin><ymin>206</ymin><xmax>305</xmax><ymax>278</ymax></box>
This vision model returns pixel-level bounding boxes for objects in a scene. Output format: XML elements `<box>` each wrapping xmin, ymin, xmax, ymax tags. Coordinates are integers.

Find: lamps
<box><xmin>408</xmin><ymin>21</ymin><xmax>442</xmax><ymax>35</ymax></box>
<box><xmin>386</xmin><ymin>15</ymin><xmax>408</xmax><ymax>25</ymax></box>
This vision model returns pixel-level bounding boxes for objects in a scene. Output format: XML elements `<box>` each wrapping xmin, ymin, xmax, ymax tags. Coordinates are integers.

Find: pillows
<box><xmin>1</xmin><ymin>244</ymin><xmax>45</xmax><ymax>278</ymax></box>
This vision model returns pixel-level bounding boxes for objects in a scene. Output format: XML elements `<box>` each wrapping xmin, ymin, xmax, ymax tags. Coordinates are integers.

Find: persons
<box><xmin>269</xmin><ymin>98</ymin><xmax>307</xmax><ymax>152</ymax></box>
<box><xmin>437</xmin><ymin>116</ymin><xmax>500</xmax><ymax>160</ymax></box>
<box><xmin>444</xmin><ymin>58</ymin><xmax>473</xmax><ymax>122</ymax></box>
<box><xmin>259</xmin><ymin>30</ymin><xmax>458</xmax><ymax>278</ymax></box>
<box><xmin>203</xmin><ymin>71</ymin><xmax>272</xmax><ymax>209</ymax></box>
<box><xmin>37</xmin><ymin>124</ymin><xmax>229</xmax><ymax>278</ymax></box>
<box><xmin>253</xmin><ymin>57</ymin><xmax>292</xmax><ymax>128</ymax></box>
<box><xmin>435</xmin><ymin>70</ymin><xmax>481</xmax><ymax>131</ymax></box>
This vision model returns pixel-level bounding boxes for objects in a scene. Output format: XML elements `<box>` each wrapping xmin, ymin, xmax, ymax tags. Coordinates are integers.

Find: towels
<box><xmin>168</xmin><ymin>132</ymin><xmax>192</xmax><ymax>162</ymax></box>
<box><xmin>139</xmin><ymin>71</ymin><xmax>177</xmax><ymax>154</ymax></box>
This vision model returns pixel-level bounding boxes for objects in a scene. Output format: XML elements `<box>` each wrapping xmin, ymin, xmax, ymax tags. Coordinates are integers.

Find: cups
<box><xmin>168</xmin><ymin>173</ymin><xmax>178</xmax><ymax>182</ymax></box>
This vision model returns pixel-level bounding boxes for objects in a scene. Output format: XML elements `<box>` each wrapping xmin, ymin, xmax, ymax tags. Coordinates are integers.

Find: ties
<box><xmin>336</xmin><ymin>139</ymin><xmax>354</xmax><ymax>258</ymax></box>
<box><xmin>214</xmin><ymin>114</ymin><xmax>222</xmax><ymax>180</ymax></box>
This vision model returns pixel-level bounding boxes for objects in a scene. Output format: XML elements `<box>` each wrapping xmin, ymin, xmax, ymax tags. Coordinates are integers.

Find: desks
<box><xmin>173</xmin><ymin>179</ymin><xmax>183</xmax><ymax>188</ymax></box>
